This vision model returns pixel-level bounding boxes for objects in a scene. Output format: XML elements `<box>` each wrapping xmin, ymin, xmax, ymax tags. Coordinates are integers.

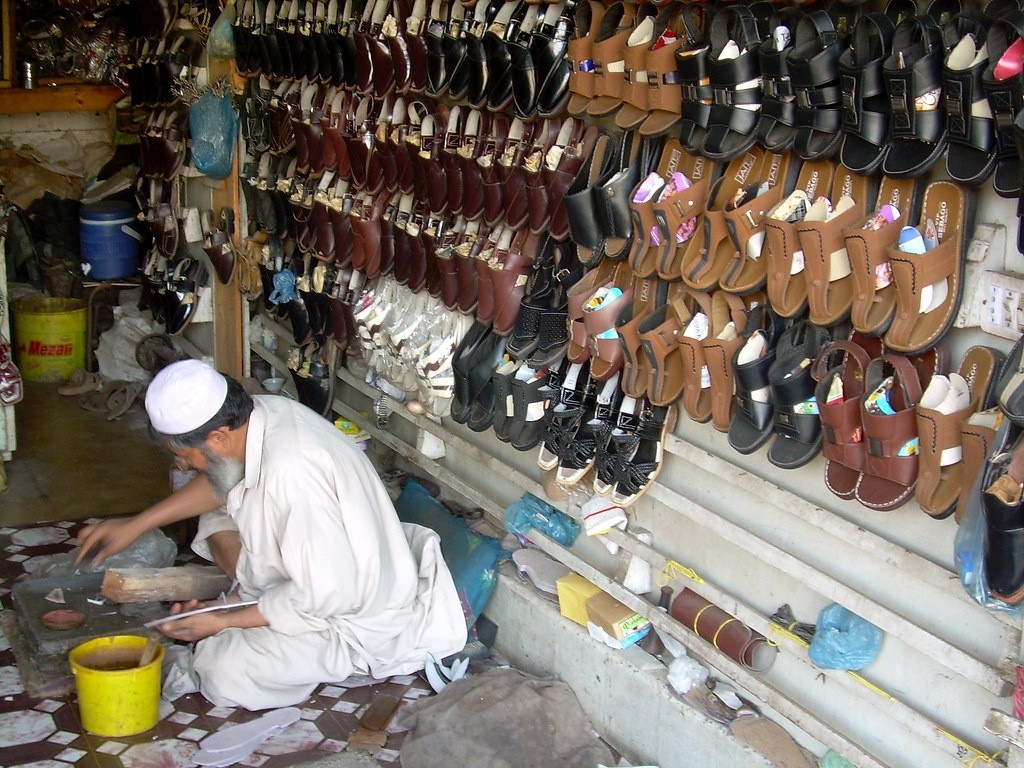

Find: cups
<box><xmin>22</xmin><ymin>60</ymin><xmax>38</xmax><ymax>90</ymax></box>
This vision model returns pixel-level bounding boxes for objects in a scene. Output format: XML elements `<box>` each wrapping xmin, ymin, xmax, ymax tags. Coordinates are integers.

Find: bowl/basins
<box><xmin>262</xmin><ymin>378</ymin><xmax>285</xmax><ymax>394</ymax></box>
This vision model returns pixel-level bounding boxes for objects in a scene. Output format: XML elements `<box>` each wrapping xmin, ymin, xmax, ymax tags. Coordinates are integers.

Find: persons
<box><xmin>74</xmin><ymin>358</ymin><xmax>468</xmax><ymax>712</ymax></box>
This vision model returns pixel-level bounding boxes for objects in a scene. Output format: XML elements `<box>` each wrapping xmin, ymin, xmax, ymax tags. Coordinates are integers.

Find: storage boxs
<box><xmin>587</xmin><ymin>592</ymin><xmax>651</xmax><ymax>637</ymax></box>
<box><xmin>335</xmin><ymin>413</ymin><xmax>367</xmax><ymax>435</ymax></box>
<box><xmin>555</xmin><ymin>572</ymin><xmax>601</xmax><ymax>628</ymax></box>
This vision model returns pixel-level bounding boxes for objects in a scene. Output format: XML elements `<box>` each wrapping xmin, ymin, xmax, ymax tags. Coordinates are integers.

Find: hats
<box><xmin>144</xmin><ymin>358</ymin><xmax>228</xmax><ymax>435</ymax></box>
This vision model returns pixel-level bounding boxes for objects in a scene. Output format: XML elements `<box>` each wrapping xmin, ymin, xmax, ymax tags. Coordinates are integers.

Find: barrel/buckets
<box><xmin>79</xmin><ymin>199</ymin><xmax>144</xmax><ymax>281</ymax></box>
<box><xmin>69</xmin><ymin>635</ymin><xmax>167</xmax><ymax>736</ymax></box>
<box><xmin>8</xmin><ymin>296</ymin><xmax>88</xmax><ymax>383</ymax></box>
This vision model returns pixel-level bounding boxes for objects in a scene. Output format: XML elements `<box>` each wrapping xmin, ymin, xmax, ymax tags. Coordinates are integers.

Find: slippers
<box><xmin>105</xmin><ymin>380</ymin><xmax>142</xmax><ymax>420</ymax></box>
<box><xmin>117</xmin><ymin>0</ymin><xmax>1024</xmax><ymax>606</ymax></box>
<box><xmin>58</xmin><ymin>368</ymin><xmax>107</xmax><ymax>396</ymax></box>
<box><xmin>76</xmin><ymin>392</ymin><xmax>112</xmax><ymax>412</ymax></box>
